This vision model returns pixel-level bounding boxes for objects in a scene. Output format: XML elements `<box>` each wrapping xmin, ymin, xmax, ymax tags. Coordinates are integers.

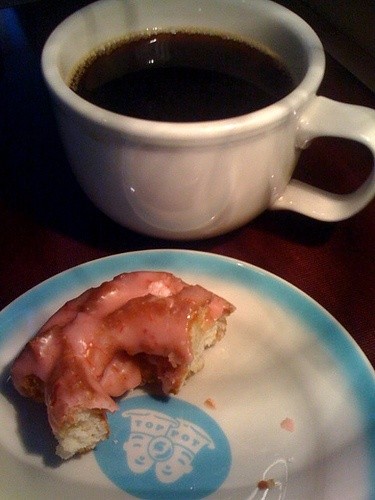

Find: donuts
<box><xmin>8</xmin><ymin>272</ymin><xmax>236</xmax><ymax>462</ymax></box>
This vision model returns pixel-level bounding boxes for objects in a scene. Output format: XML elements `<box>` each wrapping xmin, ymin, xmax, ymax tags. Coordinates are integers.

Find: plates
<box><xmin>0</xmin><ymin>247</ymin><xmax>373</xmax><ymax>495</ymax></box>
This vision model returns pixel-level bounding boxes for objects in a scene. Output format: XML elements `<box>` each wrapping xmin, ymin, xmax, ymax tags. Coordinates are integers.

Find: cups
<box><xmin>39</xmin><ymin>4</ymin><xmax>375</xmax><ymax>240</ymax></box>
